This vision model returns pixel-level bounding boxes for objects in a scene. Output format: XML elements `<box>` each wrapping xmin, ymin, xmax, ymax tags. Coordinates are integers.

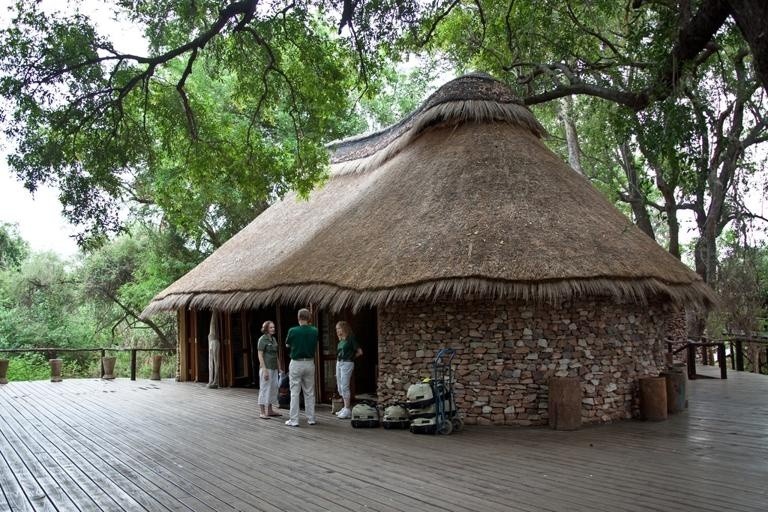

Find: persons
<box><xmin>334</xmin><ymin>320</ymin><xmax>363</xmax><ymax>419</ymax></box>
<box><xmin>281</xmin><ymin>309</ymin><xmax>319</xmax><ymax>426</ymax></box>
<box><xmin>256</xmin><ymin>320</ymin><xmax>284</xmax><ymax>420</ymax></box>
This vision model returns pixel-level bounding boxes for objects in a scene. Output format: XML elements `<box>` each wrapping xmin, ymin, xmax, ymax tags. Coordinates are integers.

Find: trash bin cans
<box><xmin>548</xmin><ymin>376</ymin><xmax>583</xmax><ymax>431</ymax></box>
<box><xmin>659</xmin><ymin>370</ymin><xmax>687</xmax><ymax>414</ymax></box>
<box><xmin>638</xmin><ymin>376</ymin><xmax>668</xmax><ymax>423</ymax></box>
<box><xmin>671</xmin><ymin>363</ymin><xmax>690</xmax><ymax>409</ymax></box>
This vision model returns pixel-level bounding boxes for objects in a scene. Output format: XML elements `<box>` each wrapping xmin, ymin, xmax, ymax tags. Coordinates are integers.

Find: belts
<box><xmin>295</xmin><ymin>358</ymin><xmax>313</xmax><ymax>360</ymax></box>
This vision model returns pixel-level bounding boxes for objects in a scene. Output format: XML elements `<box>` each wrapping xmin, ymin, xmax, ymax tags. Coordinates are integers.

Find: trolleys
<box><xmin>421</xmin><ymin>348</ymin><xmax>464</xmax><ymax>436</ymax></box>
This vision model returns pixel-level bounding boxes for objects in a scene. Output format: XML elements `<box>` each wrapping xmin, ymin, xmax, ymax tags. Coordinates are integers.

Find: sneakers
<box><xmin>335</xmin><ymin>407</ymin><xmax>352</xmax><ymax>419</ymax></box>
<box><xmin>284</xmin><ymin>419</ymin><xmax>299</xmax><ymax>427</ymax></box>
